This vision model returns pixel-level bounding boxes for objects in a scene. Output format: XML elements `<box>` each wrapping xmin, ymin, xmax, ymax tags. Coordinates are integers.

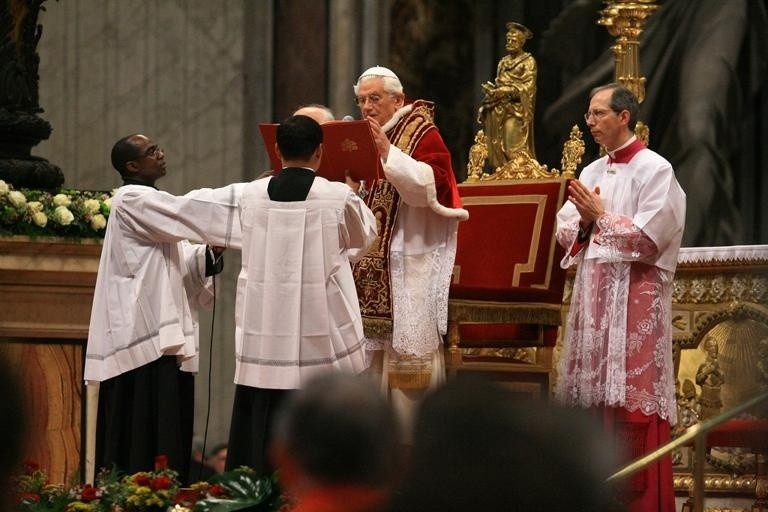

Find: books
<box><xmin>258</xmin><ymin>119</ymin><xmax>381</xmax><ymax>192</ymax></box>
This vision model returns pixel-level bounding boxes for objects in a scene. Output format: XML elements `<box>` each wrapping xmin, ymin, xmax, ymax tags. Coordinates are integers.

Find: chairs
<box><xmin>442</xmin><ymin>124</ymin><xmax>587</xmax><ymax>403</ymax></box>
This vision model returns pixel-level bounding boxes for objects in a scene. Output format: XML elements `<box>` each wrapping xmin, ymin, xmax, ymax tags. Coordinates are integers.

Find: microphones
<box><xmin>342</xmin><ymin>115</ymin><xmax>354</xmax><ymax>121</ymax></box>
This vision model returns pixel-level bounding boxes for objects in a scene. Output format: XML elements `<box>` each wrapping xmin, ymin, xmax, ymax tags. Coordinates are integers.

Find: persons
<box><xmin>261</xmin><ymin>371</ymin><xmax>627</xmax><ymax>512</ymax></box>
<box><xmin>79</xmin><ymin>132</ymin><xmax>240</xmax><ymax>486</ymax></box>
<box><xmin>222</xmin><ymin>115</ymin><xmax>375</xmax><ymax>472</ymax></box>
<box><xmin>480</xmin><ymin>23</ymin><xmax>539</xmax><ymax>170</ymax></box>
<box><xmin>294</xmin><ymin>106</ymin><xmax>337</xmax><ymax>126</ymax></box>
<box><xmin>554</xmin><ymin>83</ymin><xmax>684</xmax><ymax>512</ymax></box>
<box><xmin>353</xmin><ymin>65</ymin><xmax>470</xmax><ymax>460</ymax></box>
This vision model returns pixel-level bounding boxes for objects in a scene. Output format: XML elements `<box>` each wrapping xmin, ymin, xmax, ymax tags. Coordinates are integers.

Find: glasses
<box><xmin>584</xmin><ymin>109</ymin><xmax>613</xmax><ymax>124</ymax></box>
<box><xmin>352</xmin><ymin>94</ymin><xmax>381</xmax><ymax>106</ymax></box>
<box><xmin>130</xmin><ymin>145</ymin><xmax>161</xmax><ymax>162</ymax></box>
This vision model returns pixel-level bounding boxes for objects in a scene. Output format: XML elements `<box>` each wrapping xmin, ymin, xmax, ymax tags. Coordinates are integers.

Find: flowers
<box><xmin>0</xmin><ymin>179</ymin><xmax>117</xmax><ymax>244</ymax></box>
<box><xmin>12</xmin><ymin>455</ymin><xmax>227</xmax><ymax>511</ymax></box>
